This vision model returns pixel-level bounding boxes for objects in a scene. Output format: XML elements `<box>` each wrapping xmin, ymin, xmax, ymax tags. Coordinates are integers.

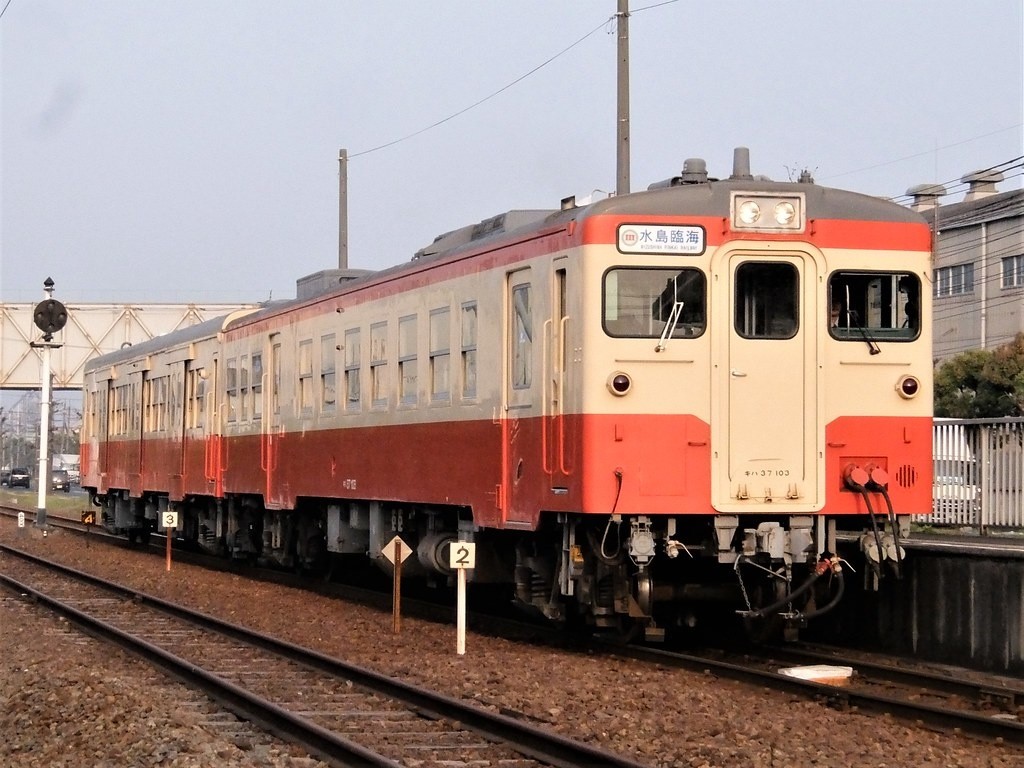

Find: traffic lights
<box><xmin>34</xmin><ymin>298</ymin><xmax>68</xmax><ymax>334</ymax></box>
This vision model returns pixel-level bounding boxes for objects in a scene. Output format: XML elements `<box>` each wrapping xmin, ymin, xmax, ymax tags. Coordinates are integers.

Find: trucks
<box><xmin>913</xmin><ymin>417</ymin><xmax>979</xmax><ymax>530</ymax></box>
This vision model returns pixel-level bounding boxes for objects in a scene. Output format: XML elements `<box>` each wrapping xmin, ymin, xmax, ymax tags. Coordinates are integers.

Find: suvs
<box><xmin>52</xmin><ymin>469</ymin><xmax>71</xmax><ymax>494</ymax></box>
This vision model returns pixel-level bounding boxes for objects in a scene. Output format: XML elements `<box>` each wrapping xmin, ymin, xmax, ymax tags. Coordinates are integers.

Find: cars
<box><xmin>0</xmin><ymin>468</ymin><xmax>31</xmax><ymax>489</ymax></box>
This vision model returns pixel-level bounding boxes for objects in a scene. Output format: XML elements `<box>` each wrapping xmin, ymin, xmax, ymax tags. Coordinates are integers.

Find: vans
<box><xmin>67</xmin><ymin>462</ymin><xmax>79</xmax><ymax>484</ymax></box>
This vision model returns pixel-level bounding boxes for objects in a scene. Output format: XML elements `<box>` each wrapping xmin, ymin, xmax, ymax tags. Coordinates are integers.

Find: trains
<box><xmin>76</xmin><ymin>146</ymin><xmax>938</xmax><ymax>648</ymax></box>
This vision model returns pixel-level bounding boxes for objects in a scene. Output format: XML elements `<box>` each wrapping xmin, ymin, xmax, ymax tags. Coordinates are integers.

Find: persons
<box><xmin>829</xmin><ymin>285</ymin><xmax>858</xmax><ymax>328</ymax></box>
